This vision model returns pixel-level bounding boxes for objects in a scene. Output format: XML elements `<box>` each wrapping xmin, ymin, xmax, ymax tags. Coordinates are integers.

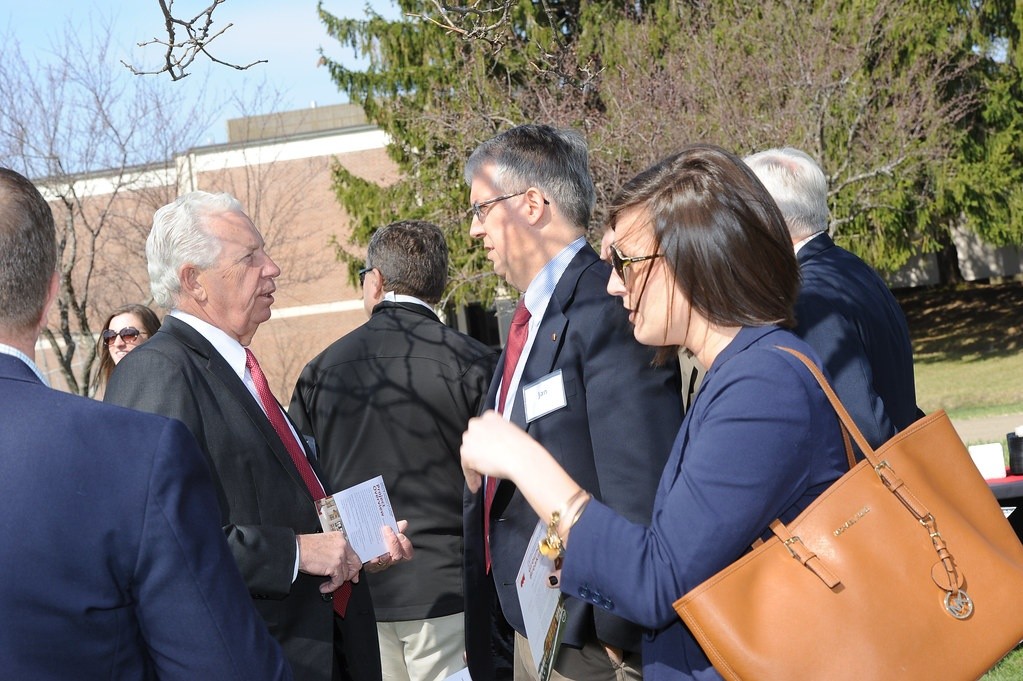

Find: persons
<box><xmin>460</xmin><ymin>145</ymin><xmax>856</xmax><ymax>681</ymax></box>
<box><xmin>0</xmin><ymin>168</ymin><xmax>294</xmax><ymax>681</ymax></box>
<box><xmin>742</xmin><ymin>148</ymin><xmax>919</xmax><ymax>465</ymax></box>
<box><xmin>104</xmin><ymin>191</ymin><xmax>414</xmax><ymax>681</ymax></box>
<box><xmin>462</xmin><ymin>123</ymin><xmax>684</xmax><ymax>681</ymax></box>
<box><xmin>287</xmin><ymin>220</ymin><xmax>500</xmax><ymax>681</ymax></box>
<box><xmin>93</xmin><ymin>304</ymin><xmax>162</xmax><ymax>402</ymax></box>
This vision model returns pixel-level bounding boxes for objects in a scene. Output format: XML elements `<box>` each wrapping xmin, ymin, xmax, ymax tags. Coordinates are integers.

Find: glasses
<box><xmin>358</xmin><ymin>266</ymin><xmax>373</xmax><ymax>288</ymax></box>
<box><xmin>607</xmin><ymin>245</ymin><xmax>664</xmax><ymax>286</ymax></box>
<box><xmin>102</xmin><ymin>327</ymin><xmax>149</xmax><ymax>346</ymax></box>
<box><xmin>464</xmin><ymin>191</ymin><xmax>550</xmax><ymax>228</ymax></box>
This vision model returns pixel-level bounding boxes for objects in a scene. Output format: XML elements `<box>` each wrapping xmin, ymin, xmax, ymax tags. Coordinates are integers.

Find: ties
<box><xmin>244</xmin><ymin>347</ymin><xmax>353</xmax><ymax>618</ymax></box>
<box><xmin>484</xmin><ymin>296</ymin><xmax>532</xmax><ymax>576</ymax></box>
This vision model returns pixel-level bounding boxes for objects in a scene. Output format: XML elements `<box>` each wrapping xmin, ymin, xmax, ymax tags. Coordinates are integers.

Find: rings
<box><xmin>378</xmin><ymin>561</ymin><xmax>388</xmax><ymax>568</ymax></box>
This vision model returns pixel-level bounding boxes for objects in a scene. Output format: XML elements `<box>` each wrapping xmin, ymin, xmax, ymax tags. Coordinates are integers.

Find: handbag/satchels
<box><xmin>670</xmin><ymin>347</ymin><xmax>1023</xmax><ymax>681</ymax></box>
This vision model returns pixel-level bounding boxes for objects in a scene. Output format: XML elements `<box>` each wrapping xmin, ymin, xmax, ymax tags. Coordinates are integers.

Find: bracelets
<box><xmin>538</xmin><ymin>490</ymin><xmax>590</xmax><ymax>560</ymax></box>
